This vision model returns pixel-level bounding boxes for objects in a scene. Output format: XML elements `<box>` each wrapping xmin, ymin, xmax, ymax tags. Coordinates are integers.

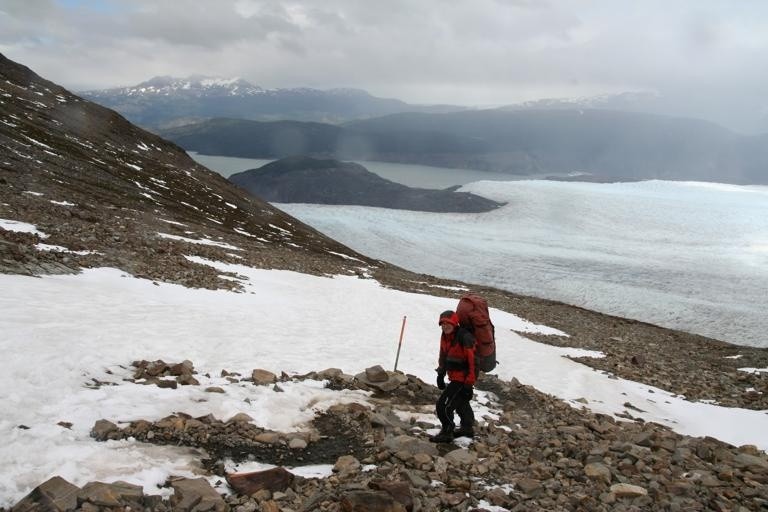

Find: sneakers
<box><xmin>454</xmin><ymin>428</ymin><xmax>474</xmax><ymax>438</ymax></box>
<box><xmin>429</xmin><ymin>433</ymin><xmax>454</xmax><ymax>443</ymax></box>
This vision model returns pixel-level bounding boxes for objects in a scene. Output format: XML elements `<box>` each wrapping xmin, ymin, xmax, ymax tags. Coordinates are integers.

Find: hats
<box><xmin>438</xmin><ymin>310</ymin><xmax>458</xmax><ymax>326</ymax></box>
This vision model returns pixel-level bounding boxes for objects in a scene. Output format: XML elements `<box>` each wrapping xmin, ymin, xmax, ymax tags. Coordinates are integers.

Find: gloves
<box><xmin>462</xmin><ymin>383</ymin><xmax>473</xmax><ymax>401</ymax></box>
<box><xmin>435</xmin><ymin>368</ymin><xmax>446</xmax><ymax>390</ymax></box>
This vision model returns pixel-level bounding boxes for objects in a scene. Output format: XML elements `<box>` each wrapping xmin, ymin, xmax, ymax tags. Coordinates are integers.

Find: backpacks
<box><xmin>454</xmin><ymin>295</ymin><xmax>497</xmax><ymax>372</ymax></box>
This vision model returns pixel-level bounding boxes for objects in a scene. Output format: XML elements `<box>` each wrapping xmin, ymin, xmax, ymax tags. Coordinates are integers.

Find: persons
<box><xmin>428</xmin><ymin>309</ymin><xmax>481</xmax><ymax>443</ymax></box>
